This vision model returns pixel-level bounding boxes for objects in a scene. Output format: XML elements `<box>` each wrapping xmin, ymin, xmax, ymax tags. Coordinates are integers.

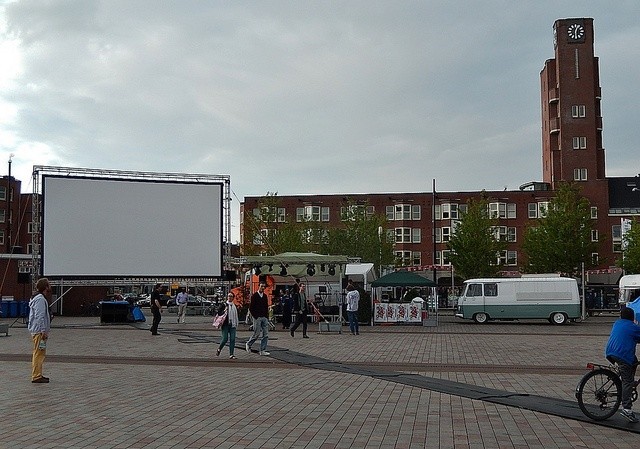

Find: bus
<box><xmin>455</xmin><ymin>276</ymin><xmax>580</xmax><ymax>326</ymax></box>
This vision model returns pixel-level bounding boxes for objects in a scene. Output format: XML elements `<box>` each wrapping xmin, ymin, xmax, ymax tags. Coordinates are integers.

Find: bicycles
<box><xmin>203</xmin><ymin>304</ymin><xmax>219</xmax><ymax>317</ymax></box>
<box><xmin>575</xmin><ymin>357</ymin><xmax>640</xmax><ymax>421</ymax></box>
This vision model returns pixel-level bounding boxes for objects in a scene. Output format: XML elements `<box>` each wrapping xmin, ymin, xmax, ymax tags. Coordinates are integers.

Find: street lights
<box><xmin>431</xmin><ymin>178</ymin><xmax>439</xmax><ymax>264</ymax></box>
<box><xmin>378</xmin><ymin>226</ymin><xmax>383</xmax><ymax>278</ymax></box>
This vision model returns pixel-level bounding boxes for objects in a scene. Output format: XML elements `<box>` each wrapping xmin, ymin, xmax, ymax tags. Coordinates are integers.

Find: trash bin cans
<box><xmin>19</xmin><ymin>301</ymin><xmax>25</xmax><ymax>318</ymax></box>
<box><xmin>9</xmin><ymin>300</ymin><xmax>19</xmax><ymax>318</ymax></box>
<box><xmin>25</xmin><ymin>300</ymin><xmax>30</xmax><ymax>318</ymax></box>
<box><xmin>0</xmin><ymin>300</ymin><xmax>8</xmax><ymax>318</ymax></box>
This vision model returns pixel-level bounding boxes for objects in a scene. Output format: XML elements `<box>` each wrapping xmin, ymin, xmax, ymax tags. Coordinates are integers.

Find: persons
<box><xmin>245</xmin><ymin>281</ymin><xmax>271</xmax><ymax>356</ymax></box>
<box><xmin>281</xmin><ymin>289</ymin><xmax>293</xmax><ymax>329</ymax></box>
<box><xmin>290</xmin><ymin>283</ymin><xmax>309</xmax><ymax>338</ymax></box>
<box><xmin>175</xmin><ymin>288</ymin><xmax>188</xmax><ymax>325</ymax></box>
<box><xmin>149</xmin><ymin>284</ymin><xmax>163</xmax><ymax>335</ymax></box>
<box><xmin>346</xmin><ymin>285</ymin><xmax>360</xmax><ymax>336</ymax></box>
<box><xmin>28</xmin><ymin>278</ymin><xmax>51</xmax><ymax>383</ymax></box>
<box><xmin>605</xmin><ymin>308</ymin><xmax>640</xmax><ymax>423</ymax></box>
<box><xmin>412</xmin><ymin>292</ymin><xmax>424</xmax><ymax>310</ymax></box>
<box><xmin>216</xmin><ymin>292</ymin><xmax>238</xmax><ymax>360</ymax></box>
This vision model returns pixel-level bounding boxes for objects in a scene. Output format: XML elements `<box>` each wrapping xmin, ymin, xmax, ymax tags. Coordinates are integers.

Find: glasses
<box><xmin>178</xmin><ymin>302</ymin><xmax>187</xmax><ymax>304</ymax></box>
<box><xmin>260</xmin><ymin>286</ymin><xmax>266</xmax><ymax>287</ymax></box>
<box><xmin>301</xmin><ymin>286</ymin><xmax>305</xmax><ymax>287</ymax></box>
<box><xmin>228</xmin><ymin>295</ymin><xmax>233</xmax><ymax>297</ymax></box>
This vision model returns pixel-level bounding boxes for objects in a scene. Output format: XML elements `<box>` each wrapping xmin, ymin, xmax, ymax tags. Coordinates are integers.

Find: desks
<box><xmin>294</xmin><ymin>314</ymin><xmax>333</xmax><ymax>324</ymax></box>
<box><xmin>273</xmin><ymin>314</ymin><xmax>294</xmax><ymax>324</ymax></box>
<box><xmin>334</xmin><ymin>315</ymin><xmax>340</xmax><ymax>323</ymax></box>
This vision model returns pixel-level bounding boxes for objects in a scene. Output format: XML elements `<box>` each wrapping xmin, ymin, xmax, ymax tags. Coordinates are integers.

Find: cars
<box><xmin>136</xmin><ymin>294</ymin><xmax>173</xmax><ymax>307</ymax></box>
<box><xmin>167</xmin><ymin>295</ymin><xmax>200</xmax><ymax>307</ymax></box>
<box><xmin>138</xmin><ymin>293</ymin><xmax>149</xmax><ymax>299</ymax></box>
<box><xmin>119</xmin><ymin>293</ymin><xmax>138</xmax><ymax>302</ymax></box>
<box><xmin>193</xmin><ymin>296</ymin><xmax>212</xmax><ymax>306</ymax></box>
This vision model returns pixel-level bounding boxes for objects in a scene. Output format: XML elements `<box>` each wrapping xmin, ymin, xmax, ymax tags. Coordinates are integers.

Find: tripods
<box><xmin>9</xmin><ymin>284</ymin><xmax>29</xmax><ymax>328</ymax></box>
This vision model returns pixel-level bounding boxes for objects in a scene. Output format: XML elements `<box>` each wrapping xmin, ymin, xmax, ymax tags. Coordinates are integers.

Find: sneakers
<box><xmin>151</xmin><ymin>333</ymin><xmax>160</xmax><ymax>335</ymax></box>
<box><xmin>149</xmin><ymin>329</ymin><xmax>153</xmax><ymax>332</ymax></box>
<box><xmin>303</xmin><ymin>335</ymin><xmax>309</xmax><ymax>338</ymax></box>
<box><xmin>286</xmin><ymin>327</ymin><xmax>289</xmax><ymax>329</ymax></box>
<box><xmin>216</xmin><ymin>349</ymin><xmax>221</xmax><ymax>356</ymax></box>
<box><xmin>260</xmin><ymin>351</ymin><xmax>270</xmax><ymax>355</ymax></box>
<box><xmin>177</xmin><ymin>318</ymin><xmax>180</xmax><ymax>323</ymax></box>
<box><xmin>282</xmin><ymin>327</ymin><xmax>285</xmax><ymax>329</ymax></box>
<box><xmin>230</xmin><ymin>355</ymin><xmax>237</xmax><ymax>360</ymax></box>
<box><xmin>32</xmin><ymin>378</ymin><xmax>49</xmax><ymax>383</ymax></box>
<box><xmin>620</xmin><ymin>409</ymin><xmax>636</xmax><ymax>422</ymax></box>
<box><xmin>291</xmin><ymin>328</ymin><xmax>294</xmax><ymax>337</ymax></box>
<box><xmin>246</xmin><ymin>343</ymin><xmax>251</xmax><ymax>354</ymax></box>
<box><xmin>181</xmin><ymin>322</ymin><xmax>185</xmax><ymax>325</ymax></box>
<box><xmin>41</xmin><ymin>376</ymin><xmax>49</xmax><ymax>380</ymax></box>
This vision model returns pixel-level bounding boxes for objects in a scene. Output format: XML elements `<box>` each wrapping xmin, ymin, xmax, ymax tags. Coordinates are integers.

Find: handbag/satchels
<box><xmin>213</xmin><ymin>303</ymin><xmax>227</xmax><ymax>329</ymax></box>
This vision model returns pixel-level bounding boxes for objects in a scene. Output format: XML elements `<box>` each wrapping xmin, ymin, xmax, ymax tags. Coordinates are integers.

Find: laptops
<box><xmin>319</xmin><ymin>286</ymin><xmax>327</xmax><ymax>292</ymax></box>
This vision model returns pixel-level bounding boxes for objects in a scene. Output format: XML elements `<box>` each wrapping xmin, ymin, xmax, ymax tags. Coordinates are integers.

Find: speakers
<box><xmin>17</xmin><ymin>272</ymin><xmax>30</xmax><ymax>283</ymax></box>
<box><xmin>217</xmin><ymin>270</ymin><xmax>237</xmax><ymax>281</ymax></box>
